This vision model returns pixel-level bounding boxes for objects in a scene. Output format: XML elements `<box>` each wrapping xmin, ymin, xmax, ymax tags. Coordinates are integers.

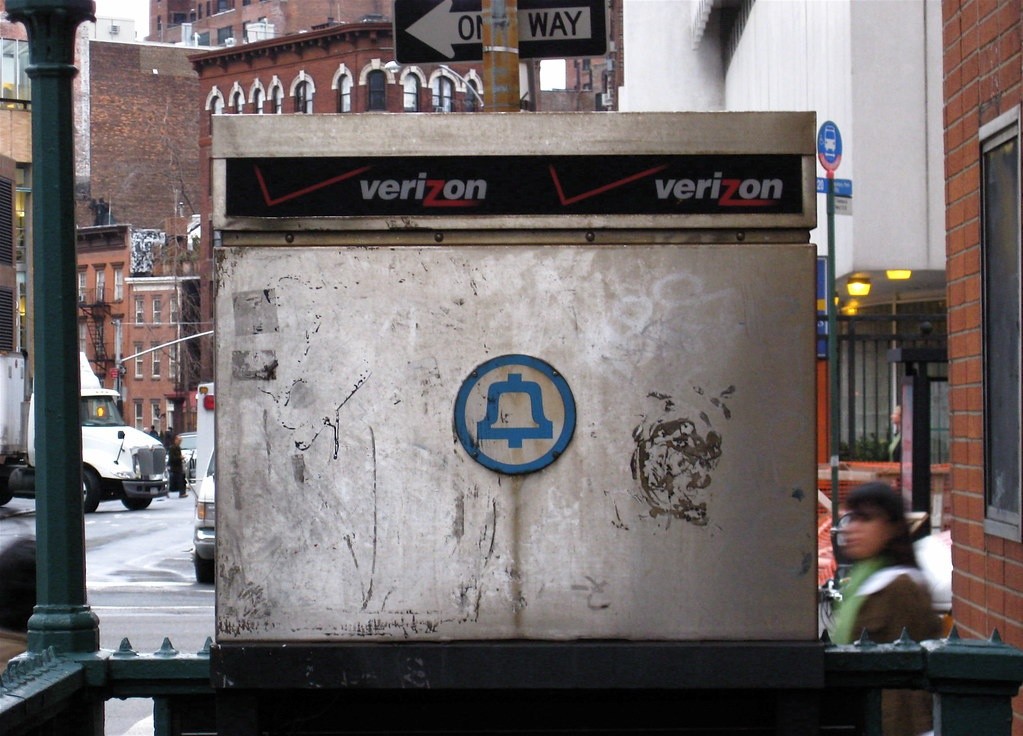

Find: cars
<box><xmin>176</xmin><ymin>432</ymin><xmax>197</xmax><ymax>481</ymax></box>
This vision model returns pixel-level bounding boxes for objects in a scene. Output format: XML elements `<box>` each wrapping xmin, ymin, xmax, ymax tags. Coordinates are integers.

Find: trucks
<box><xmin>191</xmin><ymin>382</ymin><xmax>216</xmax><ymax>586</ymax></box>
<box><xmin>0</xmin><ymin>351</ymin><xmax>171</xmax><ymax>514</ymax></box>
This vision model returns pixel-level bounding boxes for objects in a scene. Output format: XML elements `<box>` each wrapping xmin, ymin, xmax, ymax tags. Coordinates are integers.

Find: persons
<box><xmin>0</xmin><ymin>537</ymin><xmax>37</xmax><ymax>674</ymax></box>
<box><xmin>143</xmin><ymin>425</ymin><xmax>175</xmax><ymax>454</ymax></box>
<box><xmin>166</xmin><ymin>435</ymin><xmax>189</xmax><ymax>499</ymax></box>
<box><xmin>887</xmin><ymin>404</ymin><xmax>901</xmax><ymax>462</ymax></box>
<box><xmin>825</xmin><ymin>479</ymin><xmax>942</xmax><ymax>644</ymax></box>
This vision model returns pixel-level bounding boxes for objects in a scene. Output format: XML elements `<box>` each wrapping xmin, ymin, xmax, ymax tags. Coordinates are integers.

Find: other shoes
<box><xmin>180</xmin><ymin>494</ymin><xmax>188</xmax><ymax>498</ymax></box>
